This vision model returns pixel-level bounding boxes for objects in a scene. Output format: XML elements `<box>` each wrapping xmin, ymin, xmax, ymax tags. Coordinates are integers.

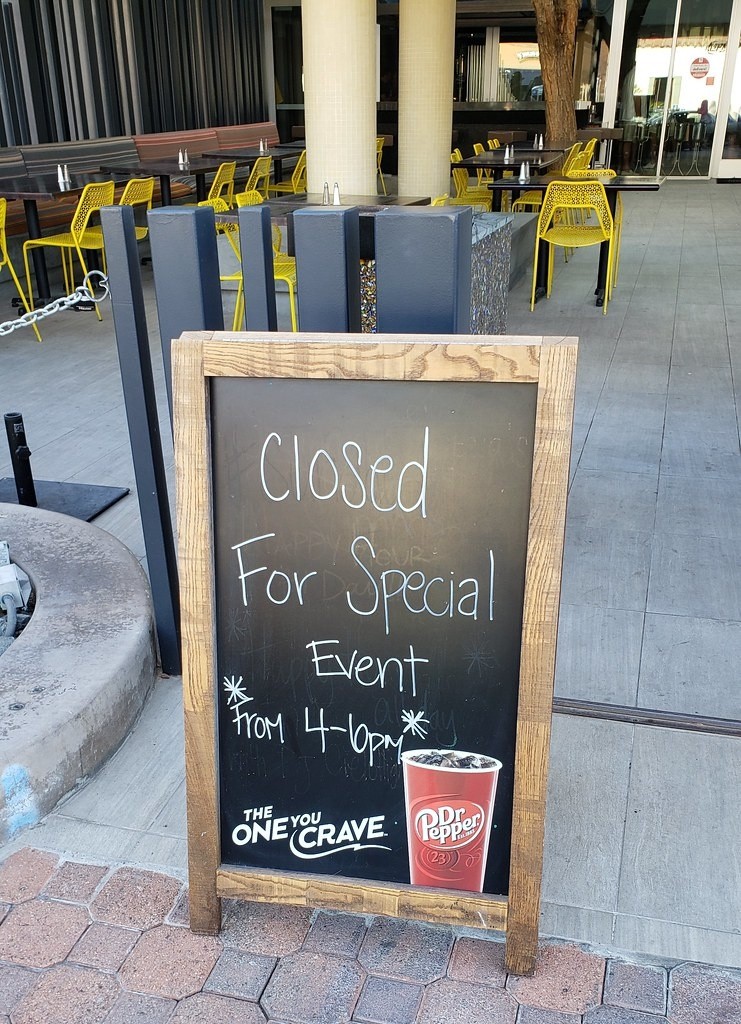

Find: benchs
<box><xmin>0</xmin><ymin>122</ymin><xmax>301</xmax><ymax>284</ymax></box>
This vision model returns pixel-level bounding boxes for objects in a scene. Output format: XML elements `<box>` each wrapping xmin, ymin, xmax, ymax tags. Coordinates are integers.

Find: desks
<box><xmin>0</xmin><ymin>172</ymin><xmax>157</xmax><ymax>316</ymax></box>
<box><xmin>202</xmin><ymin>146</ymin><xmax>304</xmax><ymax>198</ymax></box>
<box><xmin>449</xmin><ymin>151</ymin><xmax>561</xmax><ymax>212</ymax></box>
<box><xmin>489</xmin><ymin>141</ymin><xmax>581</xmax><ymax>212</ymax></box>
<box><xmin>99</xmin><ymin>158</ymin><xmax>256</xmax><ymax>265</ymax></box>
<box><xmin>270</xmin><ymin>140</ymin><xmax>306</xmax><ymax>199</ymax></box>
<box><xmin>487</xmin><ymin>176</ymin><xmax>667</xmax><ymax>307</ymax></box>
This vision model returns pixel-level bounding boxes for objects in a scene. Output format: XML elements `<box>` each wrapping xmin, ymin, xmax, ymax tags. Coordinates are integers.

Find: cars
<box><xmin>645</xmin><ymin>107</ymin><xmax>741</xmax><ymax>150</ymax></box>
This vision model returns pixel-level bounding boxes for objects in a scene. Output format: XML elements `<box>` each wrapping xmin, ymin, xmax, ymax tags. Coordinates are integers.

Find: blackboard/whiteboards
<box><xmin>163</xmin><ymin>331</ymin><xmax>582</xmax><ymax>927</ymax></box>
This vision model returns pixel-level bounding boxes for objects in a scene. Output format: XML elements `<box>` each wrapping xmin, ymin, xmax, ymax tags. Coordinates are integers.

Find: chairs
<box><xmin>0</xmin><ymin>129</ymin><xmax>622</xmax><ymax>343</ymax></box>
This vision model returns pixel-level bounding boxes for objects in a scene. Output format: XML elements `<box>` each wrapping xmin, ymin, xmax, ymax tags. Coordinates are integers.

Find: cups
<box><xmin>401</xmin><ymin>749</ymin><xmax>502</xmax><ymax>893</ymax></box>
